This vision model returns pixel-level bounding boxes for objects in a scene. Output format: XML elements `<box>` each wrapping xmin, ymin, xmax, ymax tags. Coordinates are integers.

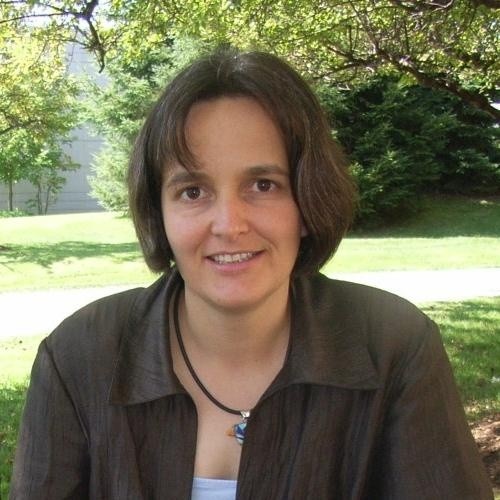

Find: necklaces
<box><xmin>173</xmin><ymin>280</ymin><xmax>293</xmax><ymax>445</ymax></box>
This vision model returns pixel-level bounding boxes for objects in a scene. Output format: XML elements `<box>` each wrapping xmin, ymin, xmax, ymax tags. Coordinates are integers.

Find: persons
<box><xmin>6</xmin><ymin>51</ymin><xmax>494</xmax><ymax>500</ymax></box>
